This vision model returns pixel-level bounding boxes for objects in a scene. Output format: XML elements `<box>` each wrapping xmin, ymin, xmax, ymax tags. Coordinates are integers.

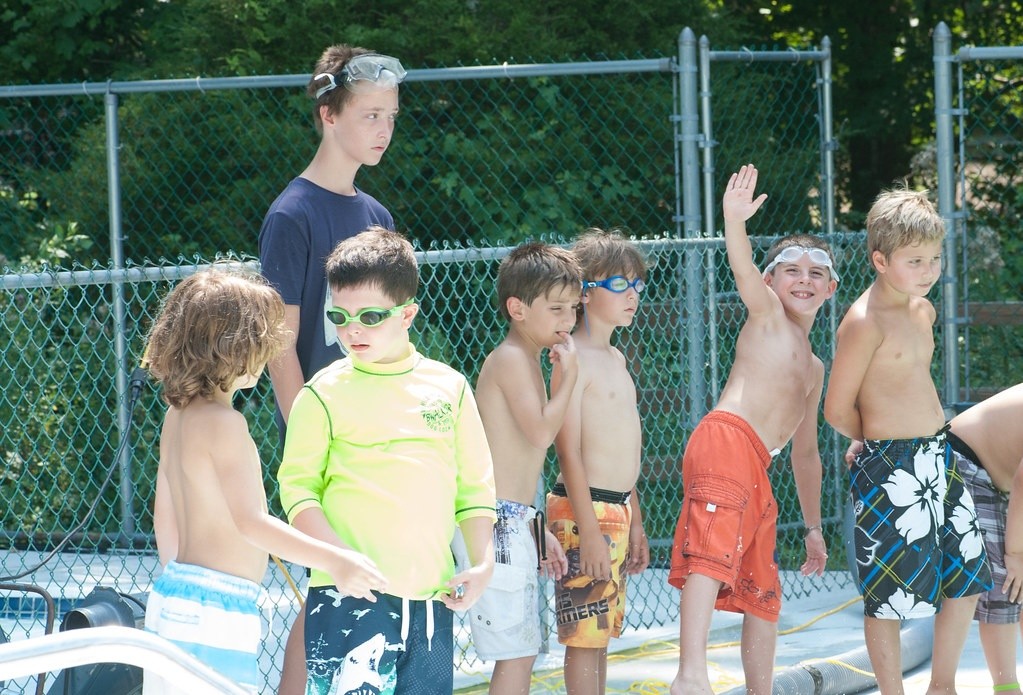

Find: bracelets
<box><xmin>797</xmin><ymin>524</ymin><xmax>823</xmax><ymax>540</ymax></box>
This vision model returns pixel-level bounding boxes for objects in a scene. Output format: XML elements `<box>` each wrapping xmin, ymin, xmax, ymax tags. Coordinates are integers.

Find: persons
<box><xmin>276</xmin><ymin>223</ymin><xmax>498</xmax><ymax>695</ymax></box>
<box><xmin>453</xmin><ymin>242</ymin><xmax>580</xmax><ymax>695</ymax></box>
<box><xmin>552</xmin><ymin>227</ymin><xmax>651</xmax><ymax>695</ymax></box>
<box><xmin>941</xmin><ymin>379</ymin><xmax>1023</xmax><ymax>694</ymax></box>
<box><xmin>143</xmin><ymin>261</ymin><xmax>391</xmax><ymax>694</ymax></box>
<box><xmin>668</xmin><ymin>165</ymin><xmax>842</xmax><ymax>695</ymax></box>
<box><xmin>823</xmin><ymin>177</ymin><xmax>995</xmax><ymax>694</ymax></box>
<box><xmin>259</xmin><ymin>43</ymin><xmax>410</xmax><ymax>695</ymax></box>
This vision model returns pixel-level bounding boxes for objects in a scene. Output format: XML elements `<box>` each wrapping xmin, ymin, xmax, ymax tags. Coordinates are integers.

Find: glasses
<box><xmin>325</xmin><ymin>298</ymin><xmax>415</xmax><ymax>326</ymax></box>
<box><xmin>762</xmin><ymin>246</ymin><xmax>841</xmax><ymax>283</ymax></box>
<box><xmin>339</xmin><ymin>54</ymin><xmax>407</xmax><ymax>95</ymax></box>
<box><xmin>585</xmin><ymin>275</ymin><xmax>646</xmax><ymax>295</ymax></box>
